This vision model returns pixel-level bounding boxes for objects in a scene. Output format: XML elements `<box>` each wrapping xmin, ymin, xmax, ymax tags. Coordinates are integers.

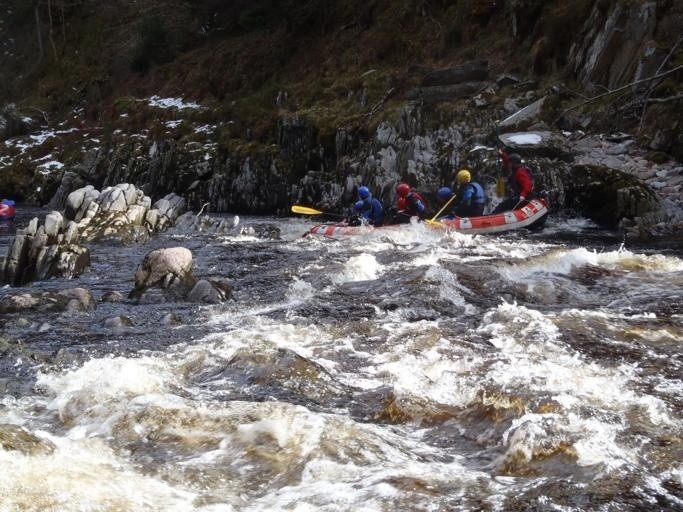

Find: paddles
<box><xmin>292</xmin><ymin>206</ymin><xmax>359</xmax><ymax>219</ymax></box>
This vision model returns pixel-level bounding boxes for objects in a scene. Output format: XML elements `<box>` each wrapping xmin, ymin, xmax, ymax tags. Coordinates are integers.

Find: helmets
<box><xmin>457</xmin><ymin>170</ymin><xmax>471</xmax><ymax>184</ymax></box>
<box><xmin>437</xmin><ymin>187</ymin><xmax>452</xmax><ymax>201</ymax></box>
<box><xmin>398</xmin><ymin>197</ymin><xmax>406</xmax><ymax>209</ymax></box>
<box><xmin>355</xmin><ymin>201</ymin><xmax>363</xmax><ymax>210</ymax></box>
<box><xmin>509</xmin><ymin>153</ymin><xmax>521</xmax><ymax>164</ymax></box>
<box><xmin>358</xmin><ymin>186</ymin><xmax>369</xmax><ymax>198</ymax></box>
<box><xmin>396</xmin><ymin>183</ymin><xmax>410</xmax><ymax>196</ymax></box>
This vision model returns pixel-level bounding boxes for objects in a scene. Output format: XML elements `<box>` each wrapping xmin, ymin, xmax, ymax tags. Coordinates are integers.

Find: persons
<box><xmin>334</xmin><ymin>144</ymin><xmax>533</xmax><ymax>229</ymax></box>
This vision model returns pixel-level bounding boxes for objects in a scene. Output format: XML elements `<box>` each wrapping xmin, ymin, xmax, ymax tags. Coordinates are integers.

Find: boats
<box><xmin>0</xmin><ymin>203</ymin><xmax>15</xmax><ymax>222</ymax></box>
<box><xmin>309</xmin><ymin>198</ymin><xmax>548</xmax><ymax>237</ymax></box>
